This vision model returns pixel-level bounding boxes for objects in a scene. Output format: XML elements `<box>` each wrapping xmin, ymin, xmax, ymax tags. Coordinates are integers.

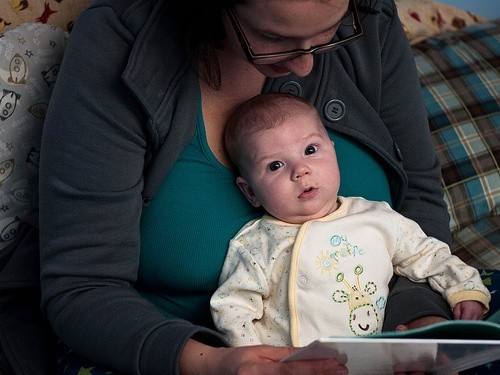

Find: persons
<box><xmin>210</xmin><ymin>92</ymin><xmax>492</xmax><ymax>347</ymax></box>
<box><xmin>38</xmin><ymin>0</ymin><xmax>500</xmax><ymax>375</ymax></box>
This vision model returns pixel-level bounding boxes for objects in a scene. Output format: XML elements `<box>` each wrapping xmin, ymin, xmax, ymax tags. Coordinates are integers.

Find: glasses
<box><xmin>226</xmin><ymin>0</ymin><xmax>363</xmax><ymax>65</ymax></box>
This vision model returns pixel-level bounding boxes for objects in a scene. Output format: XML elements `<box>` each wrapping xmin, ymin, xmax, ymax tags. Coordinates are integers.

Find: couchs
<box><xmin>0</xmin><ymin>0</ymin><xmax>500</xmax><ymax>375</ymax></box>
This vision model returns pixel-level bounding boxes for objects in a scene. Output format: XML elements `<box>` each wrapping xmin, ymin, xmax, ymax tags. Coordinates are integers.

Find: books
<box><xmin>279</xmin><ymin>309</ymin><xmax>500</xmax><ymax>375</ymax></box>
